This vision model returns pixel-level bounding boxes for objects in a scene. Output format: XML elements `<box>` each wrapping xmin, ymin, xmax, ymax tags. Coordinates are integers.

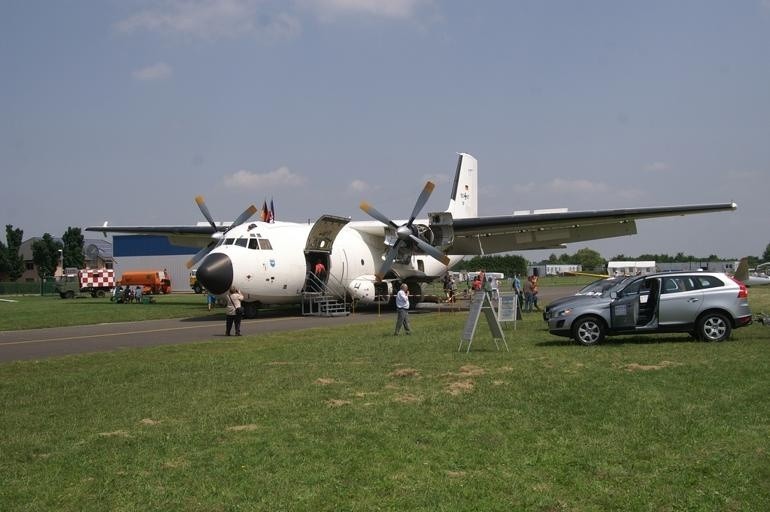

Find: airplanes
<box><xmin>82</xmin><ymin>154</ymin><xmax>740</xmax><ymax>315</ymax></box>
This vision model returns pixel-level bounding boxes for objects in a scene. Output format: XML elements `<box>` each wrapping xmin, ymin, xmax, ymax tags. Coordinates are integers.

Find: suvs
<box><xmin>548</xmin><ymin>271</ymin><xmax>755</xmax><ymax>347</ymax></box>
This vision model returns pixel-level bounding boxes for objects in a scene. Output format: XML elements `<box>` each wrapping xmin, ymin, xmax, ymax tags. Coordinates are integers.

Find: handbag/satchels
<box><xmin>236</xmin><ymin>307</ymin><xmax>244</xmax><ymax>314</ymax></box>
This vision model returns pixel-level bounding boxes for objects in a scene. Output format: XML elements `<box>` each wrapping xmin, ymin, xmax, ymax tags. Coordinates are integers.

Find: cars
<box><xmin>543</xmin><ymin>276</ymin><xmax>636</xmax><ymax>324</ymax></box>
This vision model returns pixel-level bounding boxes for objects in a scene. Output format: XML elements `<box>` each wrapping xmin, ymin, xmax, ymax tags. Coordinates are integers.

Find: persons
<box><xmin>314</xmin><ymin>259</ymin><xmax>327</xmax><ymax>292</ymax></box>
<box><xmin>441</xmin><ymin>270</ymin><xmax>538</xmax><ymax>313</ymax></box>
<box><xmin>224</xmin><ymin>286</ymin><xmax>244</xmax><ymax>336</ymax></box>
<box><xmin>204</xmin><ymin>288</ymin><xmax>216</xmax><ymax>311</ymax></box>
<box><xmin>394</xmin><ymin>283</ymin><xmax>412</xmax><ymax>336</ymax></box>
<box><xmin>115</xmin><ymin>284</ymin><xmax>142</xmax><ymax>304</ymax></box>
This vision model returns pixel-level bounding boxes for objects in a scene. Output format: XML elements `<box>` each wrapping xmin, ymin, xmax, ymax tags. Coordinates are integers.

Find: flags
<box><xmin>260</xmin><ymin>200</ymin><xmax>268</xmax><ymax>222</ymax></box>
<box><xmin>268</xmin><ymin>200</ymin><xmax>274</xmax><ymax>222</ymax></box>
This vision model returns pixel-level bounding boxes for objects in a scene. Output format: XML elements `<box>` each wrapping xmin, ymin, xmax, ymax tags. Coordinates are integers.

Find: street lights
<box><xmin>58</xmin><ymin>249</ymin><xmax>64</xmax><ymax>276</ymax></box>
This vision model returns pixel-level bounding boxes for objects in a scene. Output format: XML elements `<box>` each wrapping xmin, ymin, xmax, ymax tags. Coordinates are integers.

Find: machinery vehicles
<box><xmin>118</xmin><ymin>270</ymin><xmax>171</xmax><ymax>296</ymax></box>
<box><xmin>187</xmin><ymin>268</ymin><xmax>206</xmax><ymax>293</ymax></box>
<box><xmin>447</xmin><ymin>269</ymin><xmax>505</xmax><ymax>283</ymax></box>
<box><xmin>56</xmin><ymin>268</ymin><xmax>116</xmax><ymax>299</ymax></box>
